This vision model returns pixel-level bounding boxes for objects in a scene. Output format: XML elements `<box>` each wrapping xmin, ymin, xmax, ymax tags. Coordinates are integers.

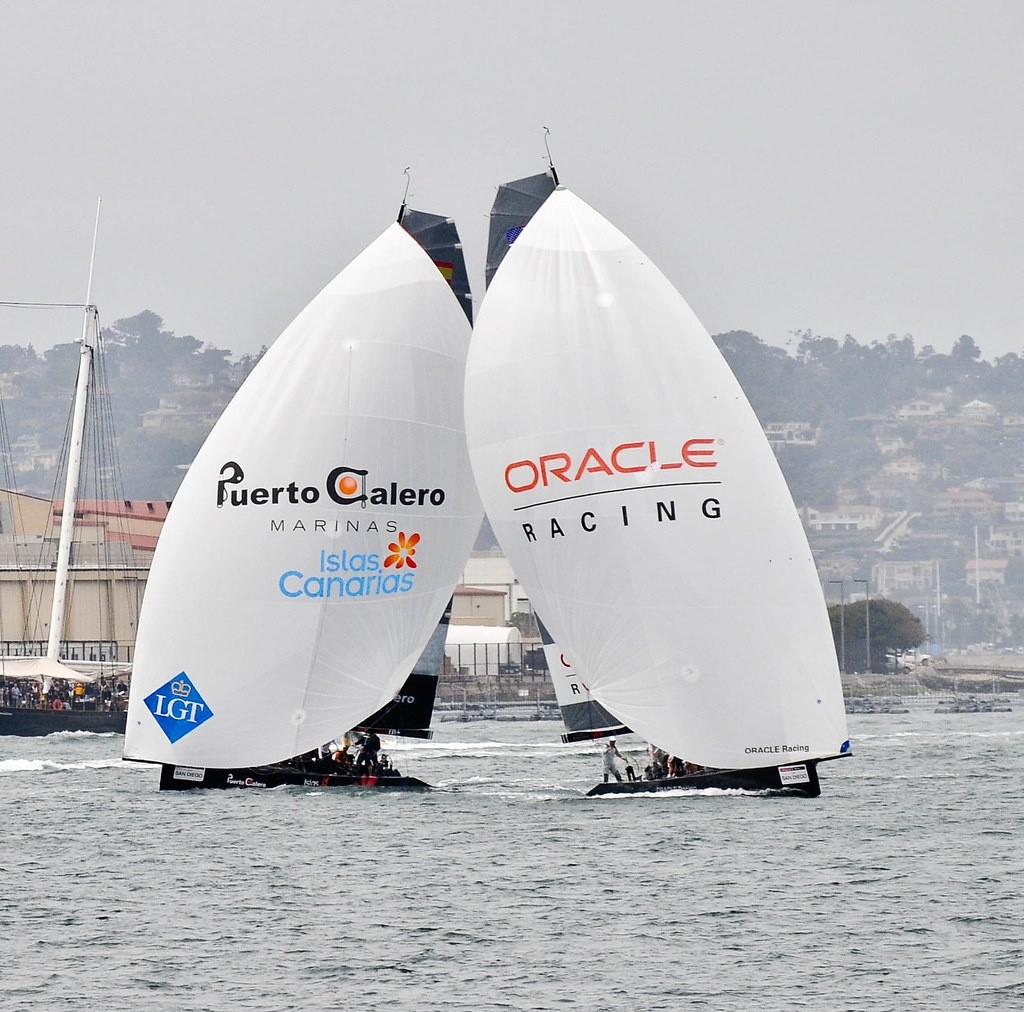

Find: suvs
<box><xmin>892</xmin><ymin>650</ymin><xmax>932</xmax><ymax>666</ymax></box>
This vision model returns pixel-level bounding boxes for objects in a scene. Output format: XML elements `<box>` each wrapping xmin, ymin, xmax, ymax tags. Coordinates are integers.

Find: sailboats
<box><xmin>464</xmin><ymin>167</ymin><xmax>852</xmax><ymax>798</ymax></box>
<box><xmin>0</xmin><ymin>197</ymin><xmax>132</xmax><ymax>737</ymax></box>
<box><xmin>122</xmin><ymin>199</ymin><xmax>485</xmax><ymax>791</ymax></box>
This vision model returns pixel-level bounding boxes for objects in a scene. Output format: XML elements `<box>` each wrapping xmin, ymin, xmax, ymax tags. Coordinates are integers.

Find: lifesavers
<box><xmin>52</xmin><ymin>699</ymin><xmax>63</xmax><ymax>710</ymax></box>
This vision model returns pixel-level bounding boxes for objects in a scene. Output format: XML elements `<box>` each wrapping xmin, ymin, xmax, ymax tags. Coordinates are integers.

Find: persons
<box><xmin>270</xmin><ymin>729</ymin><xmax>389</xmax><ymax>771</ymax></box>
<box><xmin>0</xmin><ymin>678</ymin><xmax>127</xmax><ymax>708</ymax></box>
<box><xmin>646</xmin><ymin>742</ymin><xmax>710</xmax><ymax>778</ymax></box>
<box><xmin>600</xmin><ymin>736</ymin><xmax>628</xmax><ymax>783</ymax></box>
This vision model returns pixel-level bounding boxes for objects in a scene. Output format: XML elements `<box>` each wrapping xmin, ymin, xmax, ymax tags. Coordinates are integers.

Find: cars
<box><xmin>498</xmin><ymin>665</ymin><xmax>524</xmax><ymax>675</ymax></box>
<box><xmin>883</xmin><ymin>655</ymin><xmax>916</xmax><ymax>674</ymax></box>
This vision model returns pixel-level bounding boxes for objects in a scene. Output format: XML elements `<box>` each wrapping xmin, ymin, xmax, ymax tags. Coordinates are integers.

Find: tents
<box><xmin>0</xmin><ymin>657</ymin><xmax>96</xmax><ymax>684</ymax></box>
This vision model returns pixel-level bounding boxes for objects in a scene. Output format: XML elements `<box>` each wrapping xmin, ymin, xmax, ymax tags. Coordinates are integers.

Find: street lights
<box><xmin>854</xmin><ymin>579</ymin><xmax>871</xmax><ymax>669</ymax></box>
<box><xmin>918</xmin><ymin>597</ymin><xmax>928</xmax><ymax>654</ymax></box>
<box><xmin>828</xmin><ymin>581</ymin><xmax>845</xmax><ymax>670</ymax></box>
